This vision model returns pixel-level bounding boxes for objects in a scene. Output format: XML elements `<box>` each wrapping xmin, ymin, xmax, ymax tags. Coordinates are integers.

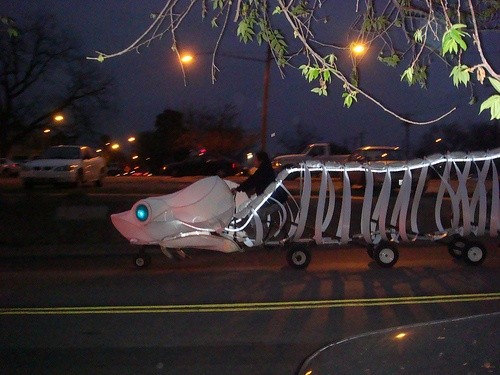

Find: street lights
<box><xmin>178</xmin><ymin>39</ymin><xmax>371</xmax><ymax>152</ymax></box>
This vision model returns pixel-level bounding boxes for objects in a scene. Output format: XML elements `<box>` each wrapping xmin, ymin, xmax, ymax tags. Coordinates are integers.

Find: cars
<box><xmin>163</xmin><ymin>153</ymin><xmax>232</xmax><ymax>179</ymax></box>
<box><xmin>340</xmin><ymin>147</ymin><xmax>408</xmax><ymax>189</ymax></box>
<box><xmin>0</xmin><ymin>158</ymin><xmax>20</xmax><ymax>175</ymax></box>
<box><xmin>21</xmin><ymin>144</ymin><xmax>107</xmax><ymax>190</ymax></box>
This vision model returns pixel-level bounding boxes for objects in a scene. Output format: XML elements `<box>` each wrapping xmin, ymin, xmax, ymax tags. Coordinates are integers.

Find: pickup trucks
<box><xmin>272</xmin><ymin>141</ymin><xmax>352</xmax><ymax>182</ymax></box>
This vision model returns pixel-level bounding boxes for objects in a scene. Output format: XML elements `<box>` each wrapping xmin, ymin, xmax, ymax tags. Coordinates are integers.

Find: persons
<box><xmin>230</xmin><ymin>152</ymin><xmax>289</xmax><ymax>240</ymax></box>
